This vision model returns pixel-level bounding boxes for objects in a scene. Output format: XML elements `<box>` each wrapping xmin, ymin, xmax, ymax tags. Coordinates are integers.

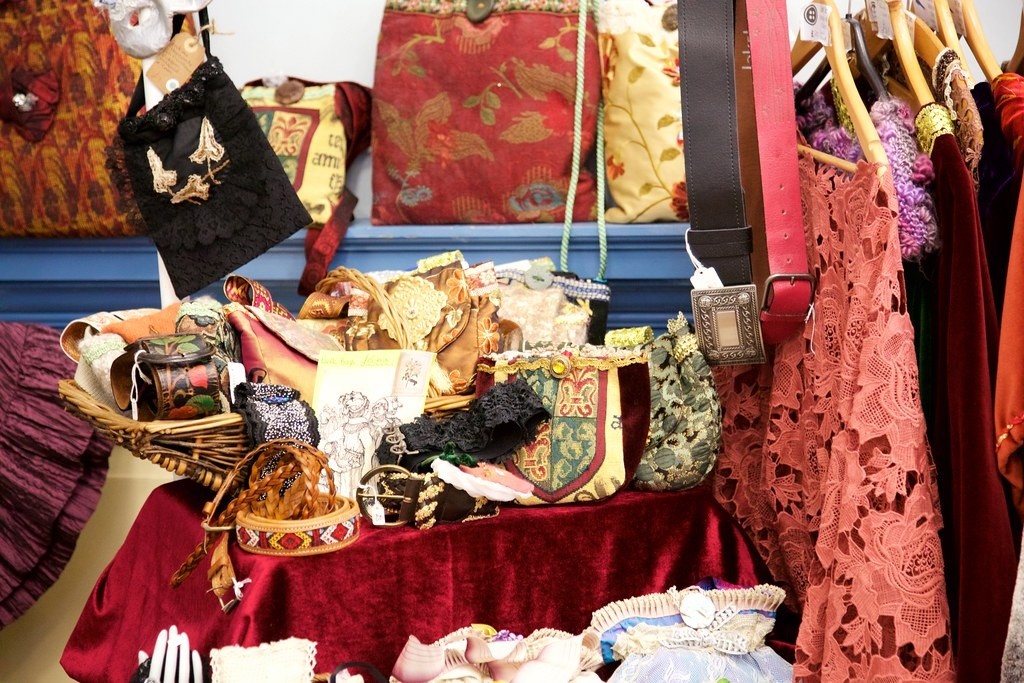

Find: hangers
<box><xmin>791</xmin><ymin>0</ymin><xmax>1002</xmax><ymax>190</ymax></box>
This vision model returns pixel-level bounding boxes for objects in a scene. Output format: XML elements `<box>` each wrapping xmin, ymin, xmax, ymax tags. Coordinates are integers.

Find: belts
<box><xmin>735</xmin><ymin>0</ymin><xmax>815</xmax><ymax>346</ymax></box>
<box><xmin>111</xmin><ymin>331</ymin><xmax>220</xmax><ymax>419</ymax></box>
<box><xmin>207</xmin><ymin>494</ymin><xmax>359</xmax><ymax>615</ymax></box>
<box><xmin>678</xmin><ymin>0</ymin><xmax>771</xmax><ymax>364</ymax></box>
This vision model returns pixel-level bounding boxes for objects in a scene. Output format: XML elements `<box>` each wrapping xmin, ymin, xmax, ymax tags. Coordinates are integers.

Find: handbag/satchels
<box><xmin>220</xmin><ymin>250</ymin><xmax>721</xmax><ymax>507</ymax></box>
<box><xmin>236</xmin><ymin>82</ymin><xmax>347</xmax><ymax>230</ymax></box>
<box><xmin>0</xmin><ymin>1</ymin><xmax>148</xmax><ymax>240</ymax></box>
<box><xmin>110</xmin><ymin>54</ymin><xmax>313</xmax><ymax>300</ymax></box>
<box><xmin>371</xmin><ymin>0</ymin><xmax>599</xmax><ymax>227</ymax></box>
<box><xmin>598</xmin><ymin>0</ymin><xmax>686</xmax><ymax>221</ymax></box>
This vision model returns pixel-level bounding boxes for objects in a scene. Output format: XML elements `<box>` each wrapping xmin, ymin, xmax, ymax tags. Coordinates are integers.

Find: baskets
<box><xmin>58</xmin><ymin>378</ymin><xmax>251</xmax><ymax>496</ymax></box>
